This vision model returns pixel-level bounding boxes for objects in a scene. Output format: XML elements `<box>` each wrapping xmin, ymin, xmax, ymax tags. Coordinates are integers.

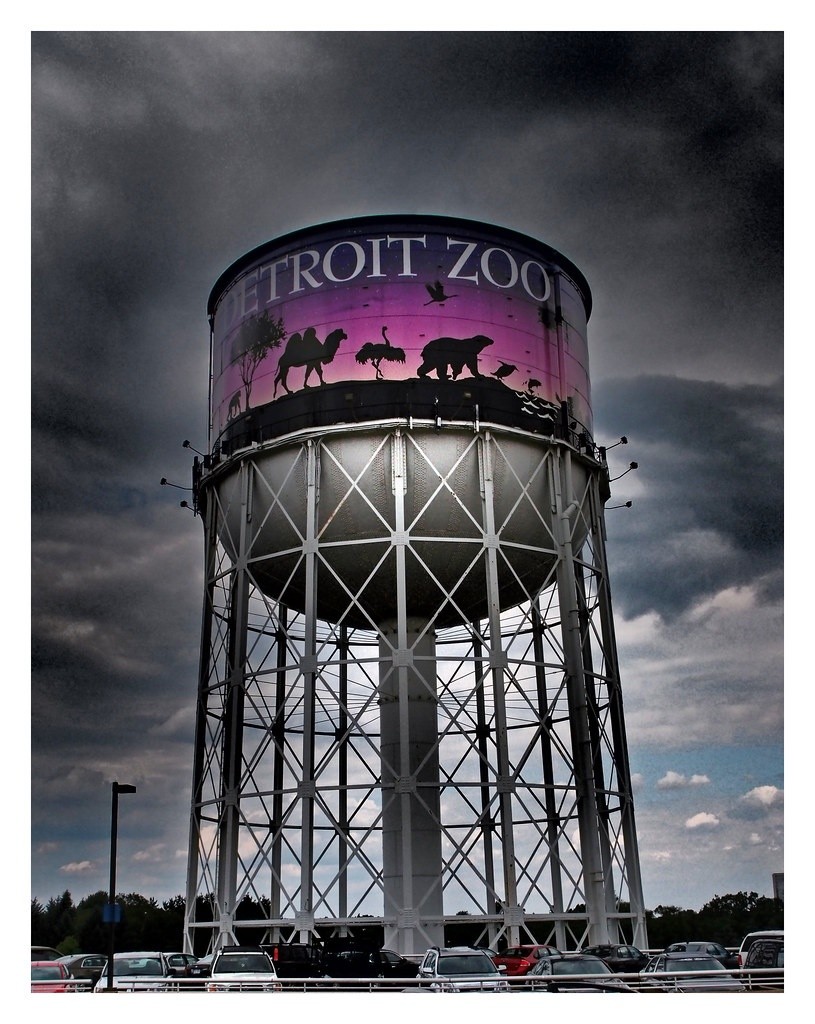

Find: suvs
<box><xmin>416</xmin><ymin>945</ymin><xmax>511</xmax><ymax>993</ymax></box>
<box><xmin>203</xmin><ymin>944</ymin><xmax>283</xmax><ymax>992</ymax></box>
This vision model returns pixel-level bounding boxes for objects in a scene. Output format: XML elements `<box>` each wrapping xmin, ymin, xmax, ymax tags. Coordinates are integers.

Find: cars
<box><xmin>491</xmin><ymin>945</ymin><xmax>564</xmax><ymax>976</ymax></box>
<box><xmin>30</xmin><ymin>946</ymin><xmax>65</xmax><ymax>963</ymax></box>
<box><xmin>580</xmin><ymin>943</ymin><xmax>651</xmax><ymax>981</ymax></box>
<box><xmin>738</xmin><ymin>930</ymin><xmax>784</xmax><ymax>990</ymax></box>
<box><xmin>183</xmin><ymin>953</ymin><xmax>215</xmax><ymax>978</ymax></box>
<box><xmin>31</xmin><ymin>961</ymin><xmax>74</xmax><ymax>993</ymax></box>
<box><xmin>662</xmin><ymin>941</ymin><xmax>740</xmax><ymax>978</ymax></box>
<box><xmin>52</xmin><ymin>953</ymin><xmax>110</xmax><ymax>992</ymax></box>
<box><xmin>130</xmin><ymin>952</ymin><xmax>200</xmax><ymax>975</ymax></box>
<box><xmin>94</xmin><ymin>952</ymin><xmax>177</xmax><ymax>992</ymax></box>
<box><xmin>369</xmin><ymin>950</ymin><xmax>422</xmax><ymax>992</ymax></box>
<box><xmin>260</xmin><ymin>943</ymin><xmax>326</xmax><ymax>980</ymax></box>
<box><xmin>526</xmin><ymin>954</ymin><xmax>638</xmax><ymax>993</ymax></box>
<box><xmin>639</xmin><ymin>952</ymin><xmax>747</xmax><ymax>993</ymax></box>
<box><xmin>481</xmin><ymin>948</ymin><xmax>498</xmax><ymax>960</ymax></box>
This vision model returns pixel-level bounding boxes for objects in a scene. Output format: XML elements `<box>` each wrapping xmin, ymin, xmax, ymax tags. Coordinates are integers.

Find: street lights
<box><xmin>104</xmin><ymin>782</ymin><xmax>138</xmax><ymax>989</ymax></box>
